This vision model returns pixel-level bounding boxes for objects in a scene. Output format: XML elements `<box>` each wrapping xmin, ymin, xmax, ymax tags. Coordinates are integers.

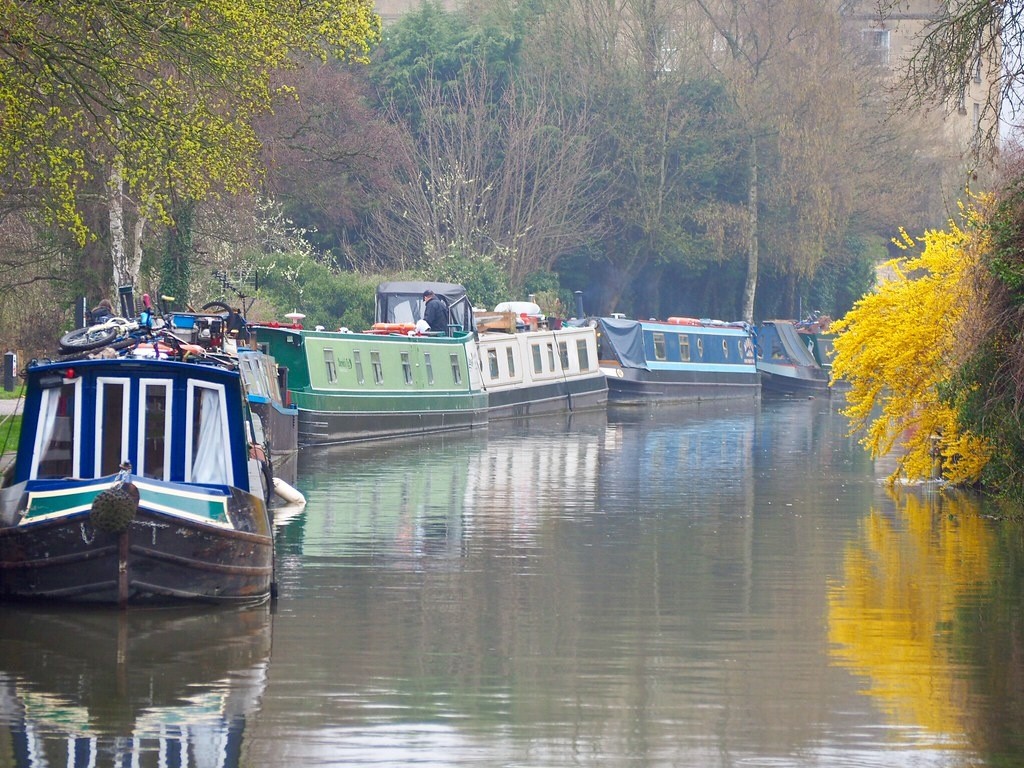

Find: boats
<box><xmin>582</xmin><ymin>314</ymin><xmax>763</xmax><ymax>406</ymax></box>
<box><xmin>756</xmin><ymin>361</ymin><xmax>829</xmax><ymax>397</ymax></box>
<box><xmin>0</xmin><ymin>279</ymin><xmax>612</xmax><ymax>610</ymax></box>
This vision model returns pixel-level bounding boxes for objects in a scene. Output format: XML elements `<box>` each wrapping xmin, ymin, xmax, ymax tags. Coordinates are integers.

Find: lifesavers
<box><xmin>372</xmin><ymin>323</ymin><xmax>416</xmax><ymax>330</ymax></box>
<box><xmin>667</xmin><ymin>316</ymin><xmax>699</xmax><ymax>326</ymax></box>
<box><xmin>130</xmin><ymin>342</ymin><xmax>204</xmax><ymax>355</ymax></box>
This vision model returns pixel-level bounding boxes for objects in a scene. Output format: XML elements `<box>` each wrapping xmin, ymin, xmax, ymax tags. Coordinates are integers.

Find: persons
<box><xmin>84</xmin><ymin>299</ymin><xmax>115</xmax><ymax>327</ymax></box>
<box><xmin>422</xmin><ymin>290</ymin><xmax>452</xmax><ymax>337</ymax></box>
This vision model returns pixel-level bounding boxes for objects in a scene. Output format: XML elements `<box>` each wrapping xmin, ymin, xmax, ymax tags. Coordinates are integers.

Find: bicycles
<box><xmin>54</xmin><ymin>293</ymin><xmax>161</xmax><ymax>363</ymax></box>
